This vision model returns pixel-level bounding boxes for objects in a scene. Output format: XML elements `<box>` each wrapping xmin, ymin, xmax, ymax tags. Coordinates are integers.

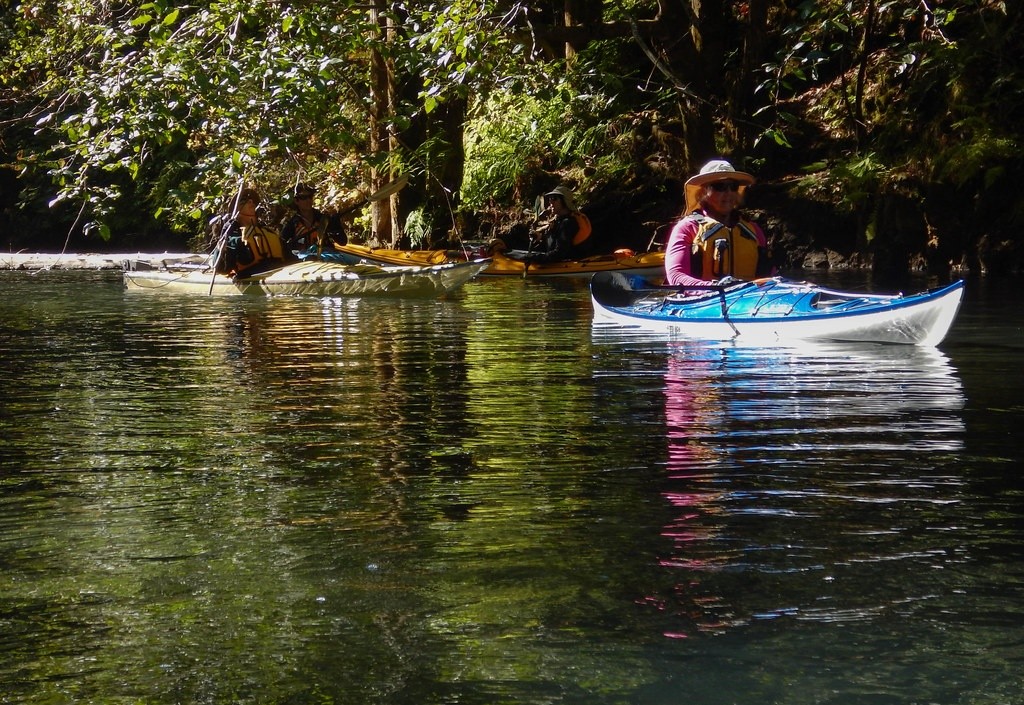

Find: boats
<box><xmin>335</xmin><ymin>236</ymin><xmax>668</xmax><ymax>296</ymax></box>
<box><xmin>120</xmin><ymin>256</ymin><xmax>493</xmax><ymax>301</ymax></box>
<box><xmin>588</xmin><ymin>269</ymin><xmax>965</xmax><ymax>348</ymax></box>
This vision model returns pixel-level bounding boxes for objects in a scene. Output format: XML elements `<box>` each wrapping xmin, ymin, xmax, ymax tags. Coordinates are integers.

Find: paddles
<box><xmin>592</xmin><ymin>269</ymin><xmax>729</xmax><ymax>309</ymax></box>
<box><xmin>208</xmin><ymin>183</ymin><xmax>245</xmax><ymax>296</ymax></box>
<box><xmin>523</xmin><ymin>196</ymin><xmax>541</xmax><ymax>279</ymax></box>
<box><xmin>289</xmin><ymin>171</ymin><xmax>412</xmax><ymax>244</ymax></box>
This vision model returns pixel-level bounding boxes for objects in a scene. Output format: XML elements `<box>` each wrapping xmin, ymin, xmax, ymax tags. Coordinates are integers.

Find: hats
<box><xmin>227</xmin><ymin>188</ymin><xmax>261</xmax><ymax>213</ymax></box>
<box><xmin>683</xmin><ymin>159</ymin><xmax>755</xmax><ymax>215</ymax></box>
<box><xmin>545</xmin><ymin>185</ymin><xmax>578</xmax><ymax>211</ymax></box>
<box><xmin>280</xmin><ymin>179</ymin><xmax>315</xmax><ymax>210</ymax></box>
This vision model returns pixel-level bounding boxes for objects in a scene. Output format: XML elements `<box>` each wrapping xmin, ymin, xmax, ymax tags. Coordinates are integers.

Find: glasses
<box><xmin>709</xmin><ymin>181</ymin><xmax>740</xmax><ymax>192</ymax></box>
<box><xmin>549</xmin><ymin>197</ymin><xmax>559</xmax><ymax>202</ymax></box>
<box><xmin>239</xmin><ymin>197</ymin><xmax>253</xmax><ymax>202</ymax></box>
<box><xmin>296</xmin><ymin>193</ymin><xmax>313</xmax><ymax>200</ymax></box>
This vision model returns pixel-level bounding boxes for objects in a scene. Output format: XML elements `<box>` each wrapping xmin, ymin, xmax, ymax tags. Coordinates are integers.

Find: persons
<box><xmin>663</xmin><ymin>158</ymin><xmax>777</xmax><ymax>297</ymax></box>
<box><xmin>524</xmin><ymin>186</ymin><xmax>592</xmax><ymax>266</ymax></box>
<box><xmin>210</xmin><ymin>182</ymin><xmax>347</xmax><ymax>279</ymax></box>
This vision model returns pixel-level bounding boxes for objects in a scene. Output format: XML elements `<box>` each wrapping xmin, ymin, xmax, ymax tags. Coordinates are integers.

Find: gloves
<box><xmin>220</xmin><ymin>219</ymin><xmax>239</xmax><ymax>239</ymax></box>
